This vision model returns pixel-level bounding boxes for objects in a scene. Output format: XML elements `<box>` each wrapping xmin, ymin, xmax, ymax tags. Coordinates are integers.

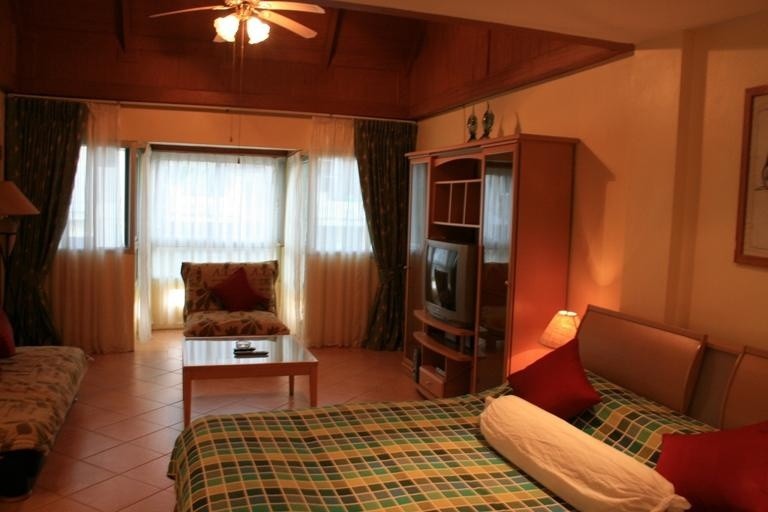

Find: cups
<box><xmin>235</xmin><ymin>341</ymin><xmax>250</xmax><ymax>348</ymax></box>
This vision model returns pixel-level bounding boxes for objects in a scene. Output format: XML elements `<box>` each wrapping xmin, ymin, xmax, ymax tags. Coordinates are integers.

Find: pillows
<box><xmin>479</xmin><ymin>395</ymin><xmax>691</xmax><ymax>512</ymax></box>
<box><xmin>507</xmin><ymin>337</ymin><xmax>603</xmax><ymax>422</ymax></box>
<box><xmin>210</xmin><ymin>266</ymin><xmax>264</xmax><ymax>312</ymax></box>
<box><xmin>0</xmin><ymin>309</ymin><xmax>17</xmax><ymax>360</ymax></box>
<box><xmin>655</xmin><ymin>421</ymin><xmax>767</xmax><ymax>512</ymax></box>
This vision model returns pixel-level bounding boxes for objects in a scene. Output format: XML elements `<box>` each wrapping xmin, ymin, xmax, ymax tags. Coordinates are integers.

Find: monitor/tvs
<box><xmin>420</xmin><ymin>239</ymin><xmax>476</xmax><ymax>327</ymax></box>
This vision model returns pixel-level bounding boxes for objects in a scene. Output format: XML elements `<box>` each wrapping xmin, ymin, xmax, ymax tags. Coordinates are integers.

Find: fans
<box><xmin>149</xmin><ymin>0</ymin><xmax>325</xmax><ymax>43</ymax></box>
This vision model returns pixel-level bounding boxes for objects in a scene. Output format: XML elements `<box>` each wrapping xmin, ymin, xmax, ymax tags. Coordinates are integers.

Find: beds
<box><xmin>166</xmin><ymin>303</ymin><xmax>768</xmax><ymax>512</ymax></box>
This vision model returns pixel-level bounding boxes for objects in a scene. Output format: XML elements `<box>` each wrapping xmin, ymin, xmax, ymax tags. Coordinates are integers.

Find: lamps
<box><xmin>0</xmin><ymin>181</ymin><xmax>40</xmax><ymax>311</ymax></box>
<box><xmin>213</xmin><ymin>10</ymin><xmax>270</xmax><ymax>164</ymax></box>
<box><xmin>537</xmin><ymin>310</ymin><xmax>581</xmax><ymax>349</ymax></box>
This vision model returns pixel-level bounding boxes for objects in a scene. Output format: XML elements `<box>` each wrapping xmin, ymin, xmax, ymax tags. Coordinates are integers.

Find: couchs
<box><xmin>181</xmin><ymin>260</ymin><xmax>290</xmax><ymax>337</ymax></box>
<box><xmin>0</xmin><ymin>346</ymin><xmax>96</xmax><ymax>503</ymax></box>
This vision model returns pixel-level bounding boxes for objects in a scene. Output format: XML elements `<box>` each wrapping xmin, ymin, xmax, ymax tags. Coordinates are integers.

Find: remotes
<box><xmin>234</xmin><ymin>347</ymin><xmax>256</xmax><ymax>351</ymax></box>
<box><xmin>234</xmin><ymin>351</ymin><xmax>269</xmax><ymax>356</ymax></box>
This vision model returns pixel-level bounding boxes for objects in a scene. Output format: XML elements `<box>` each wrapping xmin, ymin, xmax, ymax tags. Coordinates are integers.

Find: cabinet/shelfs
<box><xmin>401</xmin><ymin>133</ymin><xmax>580</xmax><ymax>401</ymax></box>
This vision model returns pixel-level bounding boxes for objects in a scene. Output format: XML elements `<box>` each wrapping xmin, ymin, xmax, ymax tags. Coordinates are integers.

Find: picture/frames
<box><xmin>733</xmin><ymin>84</ymin><xmax>768</xmax><ymax>269</ymax></box>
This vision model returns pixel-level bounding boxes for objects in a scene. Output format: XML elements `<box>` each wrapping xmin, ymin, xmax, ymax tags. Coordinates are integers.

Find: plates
<box><xmin>233</xmin><ymin>349</ymin><xmax>255</xmax><ymax>352</ymax></box>
